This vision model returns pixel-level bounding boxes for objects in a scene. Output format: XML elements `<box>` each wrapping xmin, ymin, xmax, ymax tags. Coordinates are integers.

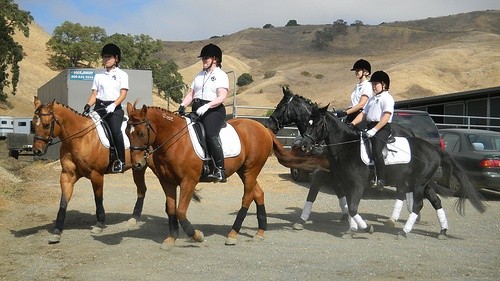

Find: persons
<box><xmin>349</xmin><ymin>70</ymin><xmax>395</xmax><ymax>191</ymax></box>
<box><xmin>84</xmin><ymin>43</ymin><xmax>129</xmax><ymax>173</ymax></box>
<box><xmin>337</xmin><ymin>58</ymin><xmax>375</xmax><ymax>131</ymax></box>
<box><xmin>178</xmin><ymin>43</ymin><xmax>230</xmax><ymax>183</ymax></box>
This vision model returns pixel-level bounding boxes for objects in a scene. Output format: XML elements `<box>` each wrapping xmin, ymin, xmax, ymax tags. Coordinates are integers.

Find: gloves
<box><xmin>365</xmin><ymin>127</ymin><xmax>377</xmax><ymax>138</ymax></box>
<box><xmin>178</xmin><ymin>105</ymin><xmax>186</xmax><ymax>115</ymax></box>
<box><xmin>105</xmin><ymin>103</ymin><xmax>116</xmax><ymax>114</ymax></box>
<box><xmin>337</xmin><ymin>111</ymin><xmax>347</xmax><ymax>118</ymax></box>
<box><xmin>196</xmin><ymin>103</ymin><xmax>210</xmax><ymax>116</ymax></box>
<box><xmin>84</xmin><ymin>104</ymin><xmax>90</xmax><ymax>113</ymax></box>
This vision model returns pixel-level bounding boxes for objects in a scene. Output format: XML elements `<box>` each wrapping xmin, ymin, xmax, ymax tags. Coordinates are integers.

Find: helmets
<box><xmin>197</xmin><ymin>43</ymin><xmax>223</xmax><ymax>63</ymax></box>
<box><xmin>350</xmin><ymin>59</ymin><xmax>371</xmax><ymax>76</ymax></box>
<box><xmin>368</xmin><ymin>70</ymin><xmax>389</xmax><ymax>90</ymax></box>
<box><xmin>100</xmin><ymin>43</ymin><xmax>122</xmax><ymax>63</ymax></box>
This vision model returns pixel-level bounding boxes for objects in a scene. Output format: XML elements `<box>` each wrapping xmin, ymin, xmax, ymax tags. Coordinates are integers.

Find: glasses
<box><xmin>199</xmin><ymin>56</ymin><xmax>211</xmax><ymax>59</ymax></box>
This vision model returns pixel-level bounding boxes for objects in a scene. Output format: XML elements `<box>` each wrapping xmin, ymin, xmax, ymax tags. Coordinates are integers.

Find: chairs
<box><xmin>472</xmin><ymin>142</ymin><xmax>484</xmax><ymax>150</ymax></box>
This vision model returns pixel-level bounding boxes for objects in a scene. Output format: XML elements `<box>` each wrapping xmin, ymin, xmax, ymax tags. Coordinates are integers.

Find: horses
<box><xmin>299</xmin><ymin>101</ymin><xmax>491</xmax><ymax>240</ymax></box>
<box><xmin>29</xmin><ymin>94</ymin><xmax>149</xmax><ymax>244</ymax></box>
<box><xmin>264</xmin><ymin>85</ymin><xmax>421</xmax><ymax>230</ymax></box>
<box><xmin>124</xmin><ymin>101</ymin><xmax>332</xmax><ymax>251</ymax></box>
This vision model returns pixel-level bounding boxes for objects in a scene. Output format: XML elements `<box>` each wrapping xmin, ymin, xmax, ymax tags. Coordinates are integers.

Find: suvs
<box><xmin>287</xmin><ymin>109</ymin><xmax>447</xmax><ymax>183</ymax></box>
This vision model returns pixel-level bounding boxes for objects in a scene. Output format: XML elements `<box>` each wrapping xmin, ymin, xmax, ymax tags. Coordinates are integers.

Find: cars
<box><xmin>438</xmin><ymin>128</ymin><xmax>500</xmax><ymax>200</ymax></box>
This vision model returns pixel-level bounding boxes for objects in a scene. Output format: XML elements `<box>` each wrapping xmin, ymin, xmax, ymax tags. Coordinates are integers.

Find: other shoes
<box><xmin>207</xmin><ymin>168</ymin><xmax>227</xmax><ymax>183</ymax></box>
<box><xmin>371</xmin><ymin>177</ymin><xmax>386</xmax><ymax>189</ymax></box>
<box><xmin>114</xmin><ymin>163</ymin><xmax>127</xmax><ymax>172</ymax></box>
